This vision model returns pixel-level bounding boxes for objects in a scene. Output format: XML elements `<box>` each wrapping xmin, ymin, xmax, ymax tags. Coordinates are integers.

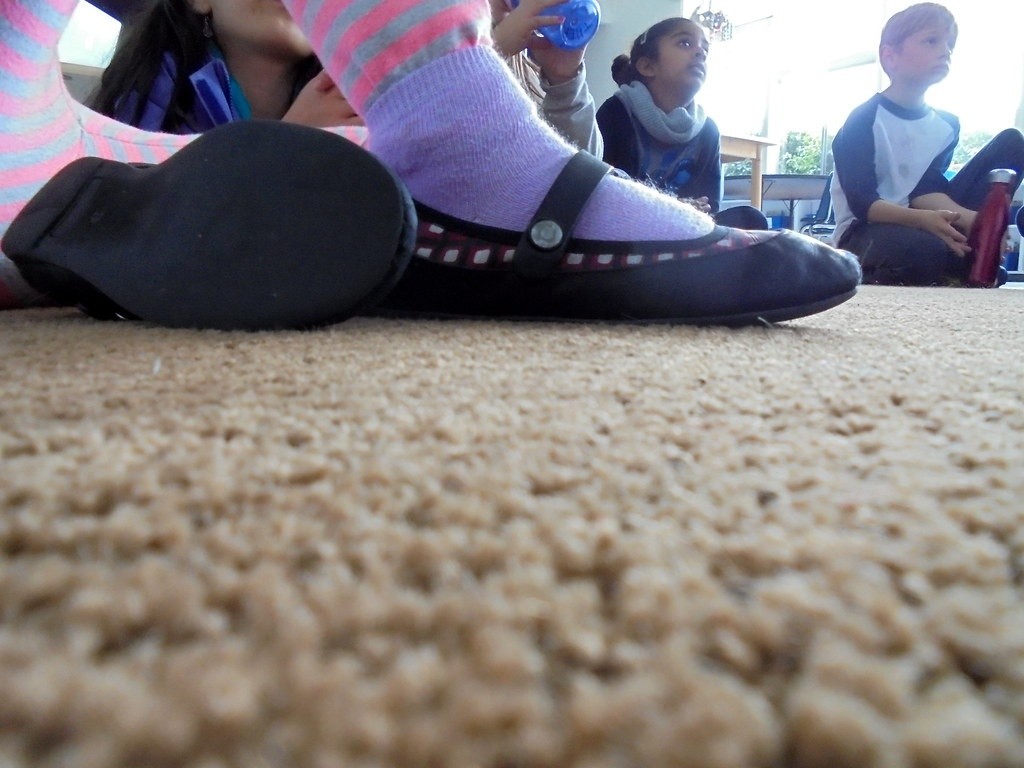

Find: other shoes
<box><xmin>0</xmin><ymin>117</ymin><xmax>418</xmax><ymax>331</ymax></box>
<box><xmin>369</xmin><ymin>148</ymin><xmax>862</xmax><ymax>324</ymax></box>
<box><xmin>921</xmin><ymin>260</ymin><xmax>1008</xmax><ymax>289</ymax></box>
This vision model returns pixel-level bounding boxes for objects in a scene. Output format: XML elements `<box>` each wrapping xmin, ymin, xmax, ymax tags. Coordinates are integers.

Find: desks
<box><xmin>720</xmin><ymin>128</ymin><xmax>777</xmax><ymax>212</ymax></box>
<box><xmin>724</xmin><ymin>173</ymin><xmax>829</xmax><ymax>228</ymax></box>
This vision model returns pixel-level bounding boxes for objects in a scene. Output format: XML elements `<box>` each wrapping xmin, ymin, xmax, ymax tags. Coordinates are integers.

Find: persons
<box><xmin>595</xmin><ymin>17</ymin><xmax>768</xmax><ymax>229</ymax></box>
<box><xmin>829</xmin><ymin>2</ymin><xmax>1024</xmax><ymax>288</ymax></box>
<box><xmin>82</xmin><ymin>0</ymin><xmax>367</xmax><ymax>136</ymax></box>
<box><xmin>0</xmin><ymin>0</ymin><xmax>859</xmax><ymax>331</ymax></box>
<box><xmin>488</xmin><ymin>0</ymin><xmax>604</xmax><ymax>161</ymax></box>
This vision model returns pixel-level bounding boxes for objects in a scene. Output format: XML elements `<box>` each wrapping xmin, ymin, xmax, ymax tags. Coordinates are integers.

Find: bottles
<box><xmin>504</xmin><ymin>0</ymin><xmax>602</xmax><ymax>51</ymax></box>
<box><xmin>966</xmin><ymin>169</ymin><xmax>1017</xmax><ymax>288</ymax></box>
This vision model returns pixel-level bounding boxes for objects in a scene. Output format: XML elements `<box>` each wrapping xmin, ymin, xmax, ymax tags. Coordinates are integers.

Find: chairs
<box><xmin>799</xmin><ymin>170</ymin><xmax>836</xmax><ymax>240</ymax></box>
